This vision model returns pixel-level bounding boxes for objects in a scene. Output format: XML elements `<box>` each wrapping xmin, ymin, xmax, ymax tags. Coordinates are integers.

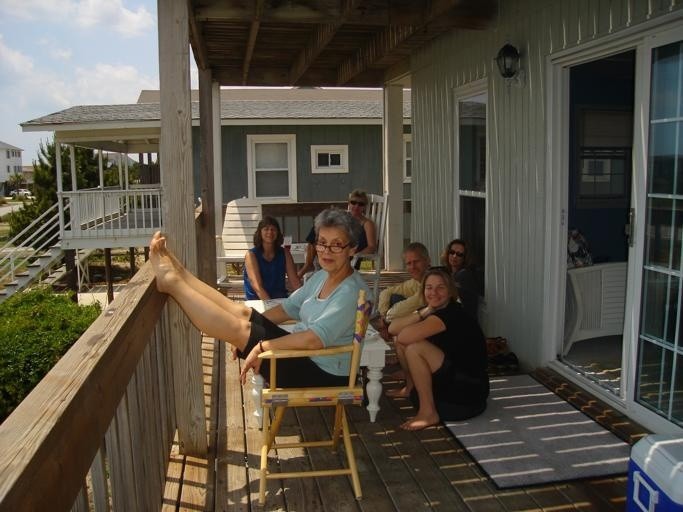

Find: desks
<box><xmin>241</xmin><ymin>298</ymin><xmax>391</xmax><ymax>428</ymax></box>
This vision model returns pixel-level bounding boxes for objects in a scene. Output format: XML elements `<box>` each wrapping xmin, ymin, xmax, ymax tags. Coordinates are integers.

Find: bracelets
<box><xmin>254</xmin><ymin>339</ymin><xmax>265</xmax><ymax>353</ymax></box>
<box><xmin>414</xmin><ymin>310</ymin><xmax>428</xmax><ymax>323</ymax></box>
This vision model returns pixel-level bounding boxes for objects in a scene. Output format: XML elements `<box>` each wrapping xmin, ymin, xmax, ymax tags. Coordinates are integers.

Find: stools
<box><xmin>255</xmin><ymin>287</ymin><xmax>368</xmax><ymax>506</ymax></box>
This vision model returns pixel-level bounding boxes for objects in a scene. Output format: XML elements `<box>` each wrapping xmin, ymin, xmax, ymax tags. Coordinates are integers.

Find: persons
<box><xmin>147</xmin><ymin>208</ymin><xmax>372</xmax><ymax>389</ymax></box>
<box><xmin>441</xmin><ymin>239</ymin><xmax>479</xmax><ymax>325</ymax></box>
<box><xmin>386</xmin><ymin>266</ymin><xmax>490</xmax><ymax>430</ymax></box>
<box><xmin>241</xmin><ymin>216</ymin><xmax>303</xmax><ymax>301</ymax></box>
<box><xmin>376</xmin><ymin>242</ymin><xmax>432</xmax><ymax>324</ymax></box>
<box><xmin>345</xmin><ymin>190</ymin><xmax>378</xmax><ymax>259</ymax></box>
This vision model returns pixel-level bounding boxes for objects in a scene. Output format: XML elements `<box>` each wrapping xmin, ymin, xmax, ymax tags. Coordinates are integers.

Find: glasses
<box><xmin>351</xmin><ymin>201</ymin><xmax>364</xmax><ymax>207</ymax></box>
<box><xmin>315</xmin><ymin>243</ymin><xmax>350</xmax><ymax>253</ymax></box>
<box><xmin>427</xmin><ymin>266</ymin><xmax>447</xmax><ymax>271</ymax></box>
<box><xmin>448</xmin><ymin>248</ymin><xmax>464</xmax><ymax>257</ymax></box>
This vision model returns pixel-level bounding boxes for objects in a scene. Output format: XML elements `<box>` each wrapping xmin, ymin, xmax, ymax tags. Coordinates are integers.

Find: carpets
<box><xmin>440</xmin><ymin>373</ymin><xmax>632</xmax><ymax>490</ymax></box>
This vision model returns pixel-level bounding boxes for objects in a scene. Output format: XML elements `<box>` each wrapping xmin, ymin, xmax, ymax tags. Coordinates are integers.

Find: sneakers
<box><xmin>501</xmin><ymin>350</ymin><xmax>520</xmax><ymax>374</ymax></box>
<box><xmin>486</xmin><ymin>352</ymin><xmax>505</xmax><ymax>372</ymax></box>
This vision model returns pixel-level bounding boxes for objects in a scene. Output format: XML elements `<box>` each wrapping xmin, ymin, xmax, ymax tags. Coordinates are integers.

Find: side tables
<box><xmin>281</xmin><ymin>243</ymin><xmax>312</xmax><ymax>264</ymax></box>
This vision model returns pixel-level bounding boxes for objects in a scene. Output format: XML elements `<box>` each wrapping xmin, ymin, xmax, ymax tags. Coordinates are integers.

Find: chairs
<box><xmin>215</xmin><ymin>197</ymin><xmax>263</xmax><ymax>288</ymax></box>
<box><xmin>346</xmin><ymin>193</ymin><xmax>390</xmax><ymax>314</ymax></box>
